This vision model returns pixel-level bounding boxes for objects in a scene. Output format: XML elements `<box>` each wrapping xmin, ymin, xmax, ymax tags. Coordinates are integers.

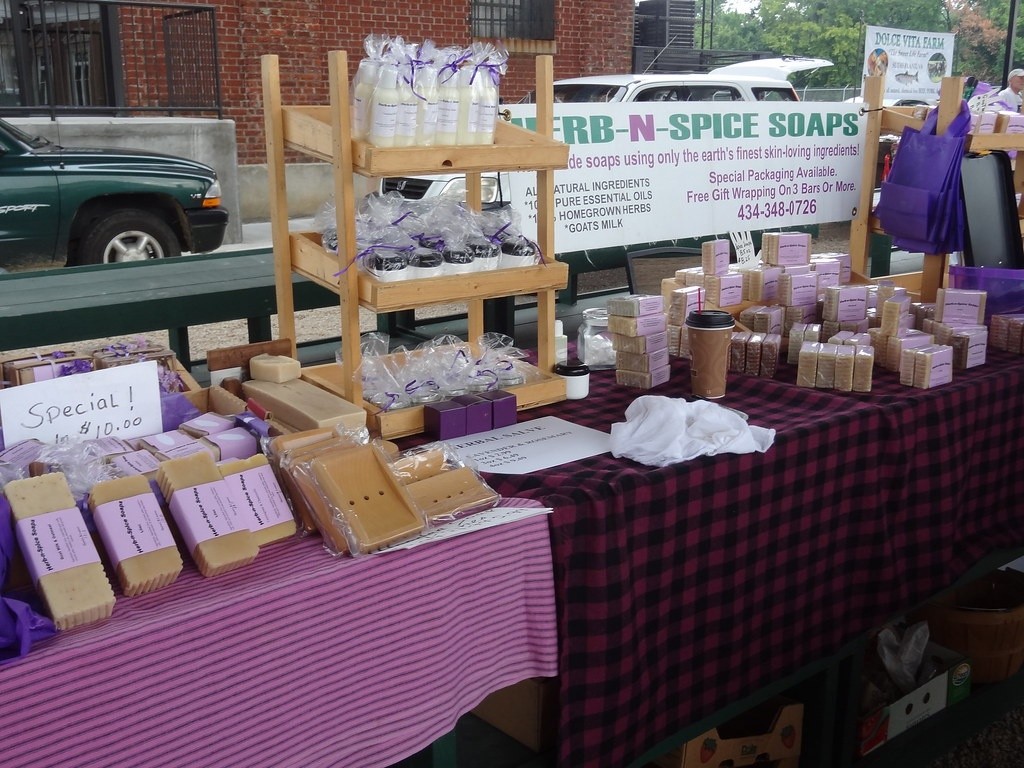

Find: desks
<box><xmin>0</xmin><ymin>337</ymin><xmax>1024</xmax><ymax>768</ymax></box>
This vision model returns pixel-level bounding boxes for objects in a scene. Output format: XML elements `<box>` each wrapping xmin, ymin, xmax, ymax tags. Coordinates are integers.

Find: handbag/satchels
<box><xmin>872</xmin><ymin>98</ymin><xmax>968</xmax><ymax>256</ymax></box>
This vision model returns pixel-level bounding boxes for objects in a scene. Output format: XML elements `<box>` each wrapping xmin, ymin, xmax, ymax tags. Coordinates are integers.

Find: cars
<box><xmin>844</xmin><ymin>94</ymin><xmax>938</xmax><ymax>108</ymax></box>
<box><xmin>1</xmin><ymin>118</ymin><xmax>230</xmax><ymax>271</ymax></box>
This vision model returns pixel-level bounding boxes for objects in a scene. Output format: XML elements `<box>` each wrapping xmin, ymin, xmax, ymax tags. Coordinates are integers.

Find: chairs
<box><xmin>625</xmin><ymin>245</ymin><xmax>703</xmax><ymax>302</ymax></box>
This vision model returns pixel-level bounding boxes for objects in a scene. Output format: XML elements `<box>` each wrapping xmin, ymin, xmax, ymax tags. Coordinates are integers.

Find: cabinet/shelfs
<box><xmin>843</xmin><ymin>73</ymin><xmax>1024</xmax><ymax>304</ymax></box>
<box><xmin>259</xmin><ymin>49</ymin><xmax>570</xmax><ymax>441</ymax></box>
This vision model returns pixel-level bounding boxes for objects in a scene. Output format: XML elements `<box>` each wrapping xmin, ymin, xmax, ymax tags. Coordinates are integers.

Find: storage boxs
<box><xmin>645</xmin><ymin>697</ymin><xmax>804</xmax><ymax>768</ymax></box>
<box><xmin>850</xmin><ymin>653</ymin><xmax>974</xmax><ymax>759</ymax></box>
<box><xmin>161</xmin><ymin>385</ymin><xmax>294</xmax><ymax>458</ymax></box>
<box><xmin>467</xmin><ymin>677</ymin><xmax>547</xmax><ymax>753</ymax></box>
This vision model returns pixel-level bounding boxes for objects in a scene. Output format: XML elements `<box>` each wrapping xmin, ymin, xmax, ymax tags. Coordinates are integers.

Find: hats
<box><xmin>1008</xmin><ymin>69</ymin><xmax>1024</xmax><ymax>82</ymax></box>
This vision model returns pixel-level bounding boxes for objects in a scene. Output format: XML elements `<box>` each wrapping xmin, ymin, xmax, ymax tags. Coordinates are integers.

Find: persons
<box><xmin>998</xmin><ymin>68</ymin><xmax>1024</xmax><ymax>112</ymax></box>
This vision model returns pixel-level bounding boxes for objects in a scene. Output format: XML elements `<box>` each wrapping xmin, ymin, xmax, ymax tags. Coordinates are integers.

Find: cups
<box><xmin>684</xmin><ymin>309</ymin><xmax>734</xmax><ymax>399</ymax></box>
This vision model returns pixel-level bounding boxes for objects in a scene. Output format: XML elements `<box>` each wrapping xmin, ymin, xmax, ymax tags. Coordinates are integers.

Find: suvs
<box><xmin>379</xmin><ymin>54</ymin><xmax>836</xmax><ymax>211</ymax></box>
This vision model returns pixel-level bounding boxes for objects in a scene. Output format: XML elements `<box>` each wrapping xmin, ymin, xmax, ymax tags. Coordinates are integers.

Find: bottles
<box><xmin>351</xmin><ymin>57</ymin><xmax>498</xmax><ymax>148</ymax></box>
<box><xmin>554</xmin><ymin>319</ymin><xmax>568</xmax><ymax>365</ymax></box>
